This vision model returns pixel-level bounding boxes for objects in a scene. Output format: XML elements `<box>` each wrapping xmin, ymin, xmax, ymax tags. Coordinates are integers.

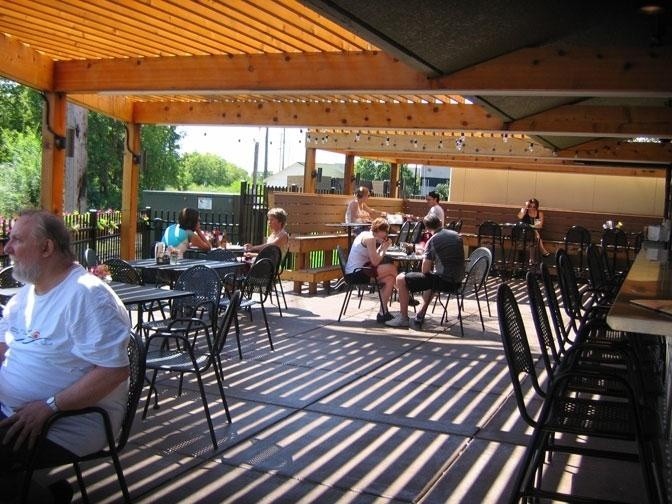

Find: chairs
<box><xmin>0</xmin><ymin>328</ymin><xmax>146</xmax><ymax>504</ymax></box>
<box><xmin>146</xmin><ymin>264</ymin><xmax>225</xmax><ymax>385</ymax></box>
<box><xmin>220</xmin><ymin>244</ymin><xmax>287</xmax><ymax>323</ymax></box>
<box><xmin>497</xmin><ymin>282</ymin><xmax>670</xmax><ymax>501</ymax></box>
<box><xmin>540</xmin><ymin>262</ymin><xmax>654</xmax><ymax>461</ymax></box>
<box><xmin>521</xmin><ymin>271</ymin><xmax>661</xmax><ymax>484</ymax></box>
<box><xmin>2</xmin><ymin>229</ymin><xmax>292</xmax><ymax>289</ymax></box>
<box><xmin>145</xmin><ymin>291</ymin><xmax>245</xmax><ymax>450</ymax></box>
<box><xmin>337</xmin><ymin>216</ymin><xmax>670</xmax><ymax>339</ymax></box>
<box><xmin>99</xmin><ymin>258</ymin><xmax>166</xmax><ymax>324</ymax></box>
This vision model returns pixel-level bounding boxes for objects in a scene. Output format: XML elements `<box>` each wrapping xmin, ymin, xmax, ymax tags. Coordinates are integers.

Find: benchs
<box><xmin>127</xmin><ymin>258</ymin><xmax>255</xmax><ymax>326</ymax></box>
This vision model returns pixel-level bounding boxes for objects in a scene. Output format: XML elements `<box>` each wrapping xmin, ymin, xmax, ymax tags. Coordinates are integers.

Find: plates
<box><xmin>385</xmin><ymin>251</ymin><xmax>407</xmax><ymax>257</ymax></box>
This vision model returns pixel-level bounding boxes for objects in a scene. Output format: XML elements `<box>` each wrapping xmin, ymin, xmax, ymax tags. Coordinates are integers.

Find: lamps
<box><xmin>351</xmin><ymin>173</ymin><xmax>360</xmax><ymax>186</ymax></box>
<box><xmin>396</xmin><ymin>180</ymin><xmax>400</xmax><ymax>187</ymax></box>
<box><xmin>56</xmin><ymin>128</ymin><xmax>75</xmax><ymax>158</ymax></box>
<box><xmin>311</xmin><ymin>168</ymin><xmax>322</xmax><ymax>181</ymax></box>
<box><xmin>133</xmin><ymin>149</ymin><xmax>147</xmax><ymax>171</ymax></box>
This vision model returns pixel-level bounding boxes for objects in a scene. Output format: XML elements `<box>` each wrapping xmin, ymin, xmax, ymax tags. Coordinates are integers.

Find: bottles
<box><xmin>212</xmin><ymin>227</ymin><xmax>227</xmax><ymax>248</ymax></box>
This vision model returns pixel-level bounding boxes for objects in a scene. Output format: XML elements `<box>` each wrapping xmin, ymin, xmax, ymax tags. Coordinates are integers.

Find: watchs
<box><xmin>46</xmin><ymin>396</ymin><xmax>60</xmax><ymax>413</ymax></box>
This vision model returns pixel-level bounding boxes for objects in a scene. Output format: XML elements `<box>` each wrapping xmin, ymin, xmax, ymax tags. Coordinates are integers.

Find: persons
<box><xmin>344</xmin><ymin>217</ymin><xmax>419</xmax><ymax>322</ymax></box>
<box><xmin>384</xmin><ymin>214</ymin><xmax>465</xmax><ymax>330</ymax></box>
<box><xmin>518</xmin><ymin>198</ymin><xmax>551</xmax><ymax>266</ymax></box>
<box><xmin>422</xmin><ymin>191</ymin><xmax>444</xmax><ymax>242</ymax></box>
<box><xmin>160</xmin><ymin>207</ymin><xmax>211</xmax><ymax>260</ymax></box>
<box><xmin>345</xmin><ymin>186</ymin><xmax>381</xmax><ymax>235</ymax></box>
<box><xmin>219</xmin><ymin>207</ymin><xmax>289</xmax><ymax>307</ymax></box>
<box><xmin>0</xmin><ymin>210</ymin><xmax>132</xmax><ymax>504</ymax></box>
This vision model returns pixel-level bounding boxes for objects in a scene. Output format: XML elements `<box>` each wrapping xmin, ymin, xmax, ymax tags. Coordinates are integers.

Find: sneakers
<box><xmin>377</xmin><ymin>295</ymin><xmax>424</xmax><ymax>327</ymax></box>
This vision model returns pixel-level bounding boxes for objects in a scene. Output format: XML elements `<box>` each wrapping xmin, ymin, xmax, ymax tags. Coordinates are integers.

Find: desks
<box><xmin>325</xmin><ymin>223</ymin><xmax>373</xmax><ymax>252</ymax></box>
<box><xmin>186</xmin><ymin>258</ymin><xmax>279</xmax><ymax>360</ymax></box>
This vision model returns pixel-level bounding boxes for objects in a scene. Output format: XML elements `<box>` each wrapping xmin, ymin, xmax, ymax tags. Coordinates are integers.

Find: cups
<box><xmin>170</xmin><ymin>252</ymin><xmax>178</xmax><ymax>265</ymax></box>
<box><xmin>415</xmin><ymin>244</ymin><xmax>423</xmax><ymax>256</ymax></box>
<box><xmin>533</xmin><ymin>217</ymin><xmax>541</xmax><ymax>228</ymax></box>
